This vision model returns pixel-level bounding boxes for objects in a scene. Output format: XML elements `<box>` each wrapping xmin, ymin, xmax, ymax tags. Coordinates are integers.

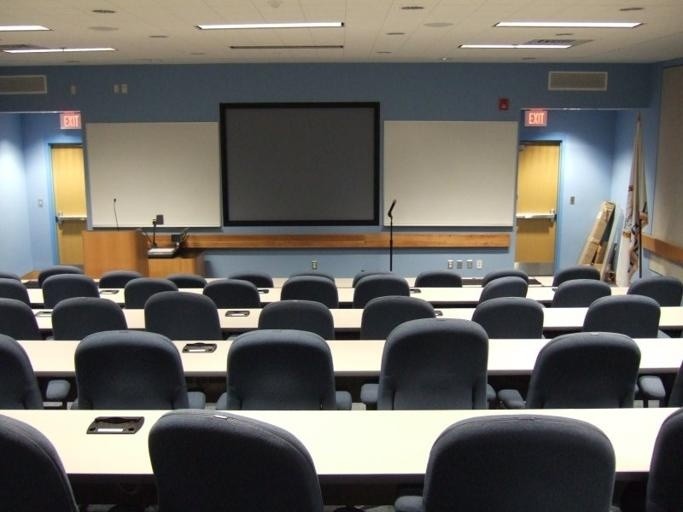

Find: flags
<box><xmin>616</xmin><ymin>120</ymin><xmax>648</xmax><ymax>287</ymax></box>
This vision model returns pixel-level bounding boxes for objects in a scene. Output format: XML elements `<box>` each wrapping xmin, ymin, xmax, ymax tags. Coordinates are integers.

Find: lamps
<box><xmin>0</xmin><ymin>46</ymin><xmax>118</xmax><ymax>54</ymax></box>
<box><xmin>0</xmin><ymin>23</ymin><xmax>51</xmax><ymax>33</ymax></box>
<box><xmin>492</xmin><ymin>20</ymin><xmax>644</xmax><ymax>30</ymax></box>
<box><xmin>458</xmin><ymin>42</ymin><xmax>574</xmax><ymax>49</ymax></box>
<box><xmin>194</xmin><ymin>20</ymin><xmax>343</xmax><ymax>30</ymax></box>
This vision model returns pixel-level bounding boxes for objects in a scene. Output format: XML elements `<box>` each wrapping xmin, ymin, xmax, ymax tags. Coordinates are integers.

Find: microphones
<box><xmin>387</xmin><ymin>200</ymin><xmax>397</xmax><ymax>216</ymax></box>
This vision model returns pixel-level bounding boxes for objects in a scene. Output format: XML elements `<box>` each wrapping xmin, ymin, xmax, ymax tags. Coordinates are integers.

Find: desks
<box><xmin>27</xmin><ymin>285</ymin><xmax>631</xmax><ymax>306</ymax></box>
<box><xmin>15</xmin><ymin>337</ymin><xmax>682</xmax><ymax>411</ymax></box>
<box><xmin>20</xmin><ymin>273</ymin><xmax>554</xmax><ymax>287</ymax></box>
<box><xmin>31</xmin><ymin>306</ymin><xmax>682</xmax><ymax>339</ymax></box>
<box><xmin>0</xmin><ymin>409</ymin><xmax>683</xmax><ymax>512</ymax></box>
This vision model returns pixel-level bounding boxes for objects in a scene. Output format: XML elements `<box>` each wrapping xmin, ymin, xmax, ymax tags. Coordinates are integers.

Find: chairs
<box><xmin>148</xmin><ymin>408</ymin><xmax>338</xmax><ymax>512</ymax></box>
<box><xmin>359</xmin><ymin>294</ymin><xmax>437</xmax><ymax>340</ymax></box>
<box><xmin>470</xmin><ymin>295</ymin><xmax>545</xmax><ymax>340</ymax></box>
<box><xmin>257</xmin><ymin>300</ymin><xmax>335</xmax><ymax>340</ymax></box>
<box><xmin>644</xmin><ymin>409</ymin><xmax>683</xmax><ymax>512</ymax></box>
<box><xmin>100</xmin><ymin>268</ymin><xmax>141</xmax><ymax>288</ymax></box>
<box><xmin>0</xmin><ymin>415</ymin><xmax>116</xmax><ymax>512</ymax></box>
<box><xmin>413</xmin><ymin>269</ymin><xmax>461</xmax><ymax>288</ymax></box>
<box><xmin>201</xmin><ymin>278</ymin><xmax>260</xmax><ymax>310</ymax></box>
<box><xmin>582</xmin><ymin>296</ymin><xmax>660</xmax><ymax>340</ymax></box>
<box><xmin>217</xmin><ymin>327</ymin><xmax>350</xmax><ymax>411</ymax></box>
<box><xmin>65</xmin><ymin>328</ymin><xmax>205</xmax><ymax>410</ymax></box>
<box><xmin>479</xmin><ymin>276</ymin><xmax>526</xmax><ymax>301</ymax></box>
<box><xmin>498</xmin><ymin>331</ymin><xmax>640</xmax><ymax>409</ymax></box>
<box><xmin>0</xmin><ymin>277</ymin><xmax>30</xmax><ymax>306</ymax></box>
<box><xmin>37</xmin><ymin>265</ymin><xmax>83</xmax><ymax>288</ymax></box>
<box><xmin>287</xmin><ymin>273</ymin><xmax>337</xmax><ymax>285</ymax></box>
<box><xmin>280</xmin><ymin>277</ymin><xmax>338</xmax><ymax>309</ymax></box>
<box><xmin>43</xmin><ymin>273</ymin><xmax>100</xmax><ymax>309</ymax></box>
<box><xmin>639</xmin><ymin>361</ymin><xmax>682</xmax><ymax>407</ymax></box>
<box><xmin>393</xmin><ymin>413</ymin><xmax>619</xmax><ymax>511</ymax></box>
<box><xmin>352</xmin><ymin>269</ymin><xmax>393</xmax><ymax>288</ymax></box>
<box><xmin>360</xmin><ymin>318</ymin><xmax>495</xmax><ymax>410</ymax></box>
<box><xmin>228</xmin><ymin>271</ymin><xmax>274</xmax><ymax>288</ymax></box>
<box><xmin>46</xmin><ymin>296</ymin><xmax>127</xmax><ymax>341</ymax></box>
<box><xmin>480</xmin><ymin>270</ymin><xmax>528</xmax><ymax>289</ymax></box>
<box><xmin>550</xmin><ymin>264</ymin><xmax>601</xmax><ymax>289</ymax></box>
<box><xmin>143</xmin><ymin>290</ymin><xmax>242</xmax><ymax>343</ymax></box>
<box><xmin>0</xmin><ymin>334</ymin><xmax>70</xmax><ymax>411</ymax></box>
<box><xmin>353</xmin><ymin>276</ymin><xmax>411</xmax><ymax>309</ymax></box>
<box><xmin>0</xmin><ymin>271</ymin><xmax>20</xmax><ymax>285</ymax></box>
<box><xmin>0</xmin><ymin>298</ymin><xmax>42</xmax><ymax>341</ymax></box>
<box><xmin>125</xmin><ymin>277</ymin><xmax>180</xmax><ymax>309</ymax></box>
<box><xmin>627</xmin><ymin>275</ymin><xmax>682</xmax><ymax>308</ymax></box>
<box><xmin>167</xmin><ymin>273</ymin><xmax>205</xmax><ymax>288</ymax></box>
<box><xmin>552</xmin><ymin>280</ymin><xmax>613</xmax><ymax>307</ymax></box>
